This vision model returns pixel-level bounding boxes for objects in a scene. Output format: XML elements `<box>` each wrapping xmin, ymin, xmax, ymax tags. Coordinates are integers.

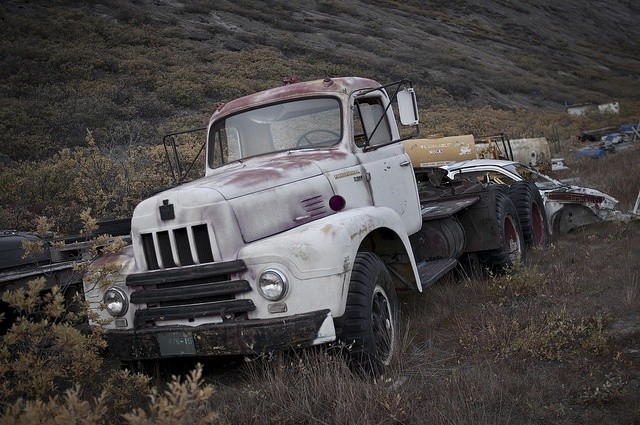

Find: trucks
<box><xmin>81</xmin><ymin>76</ymin><xmax>550</xmax><ymax>381</ymax></box>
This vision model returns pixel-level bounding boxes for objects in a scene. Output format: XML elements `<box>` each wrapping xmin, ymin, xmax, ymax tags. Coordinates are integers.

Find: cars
<box><xmin>436</xmin><ymin>158</ymin><xmax>620</xmax><ymax>236</ymax></box>
